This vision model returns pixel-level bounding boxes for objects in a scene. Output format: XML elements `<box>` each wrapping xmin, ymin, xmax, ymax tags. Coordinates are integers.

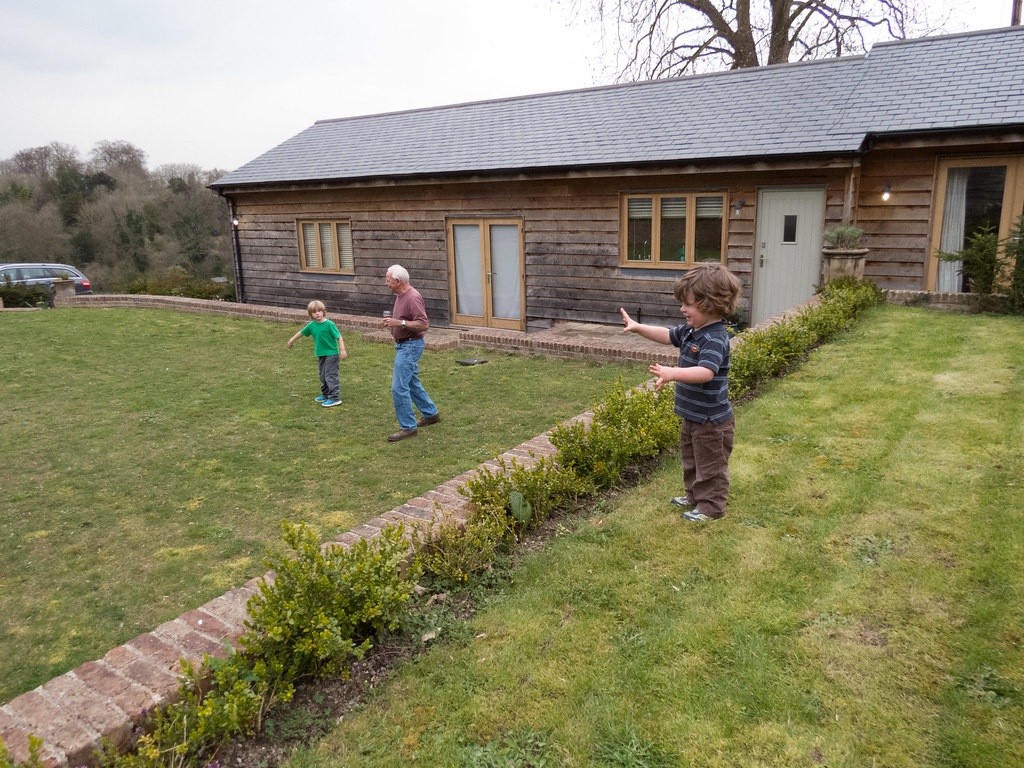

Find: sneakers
<box><xmin>671</xmin><ymin>496</ymin><xmax>693</xmax><ymax>508</ymax></box>
<box><xmin>683</xmin><ymin>509</ymin><xmax>716</xmax><ymax>523</ymax></box>
<box><xmin>315</xmin><ymin>395</ymin><xmax>328</xmax><ymax>403</ymax></box>
<box><xmin>417</xmin><ymin>413</ymin><xmax>440</xmax><ymax>427</ymax></box>
<box><xmin>322</xmin><ymin>398</ymin><xmax>342</xmax><ymax>406</ymax></box>
<box><xmin>387</xmin><ymin>428</ymin><xmax>417</xmax><ymax>441</ymax></box>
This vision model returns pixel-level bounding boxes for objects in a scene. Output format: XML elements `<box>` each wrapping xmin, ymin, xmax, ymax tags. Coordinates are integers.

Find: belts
<box><xmin>396</xmin><ymin>336</ymin><xmax>421</xmax><ymax>343</ymax></box>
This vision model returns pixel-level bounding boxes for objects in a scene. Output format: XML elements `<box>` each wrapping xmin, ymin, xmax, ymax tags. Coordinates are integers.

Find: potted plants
<box><xmin>822</xmin><ymin>225</ymin><xmax>870</xmax><ymax>285</ymax></box>
<box><xmin>52</xmin><ymin>271</ymin><xmax>75</xmax><ymax>297</ymax></box>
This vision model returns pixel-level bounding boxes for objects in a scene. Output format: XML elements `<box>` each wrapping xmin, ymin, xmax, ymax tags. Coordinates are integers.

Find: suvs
<box><xmin>0</xmin><ymin>263</ymin><xmax>94</xmax><ymax>308</ymax></box>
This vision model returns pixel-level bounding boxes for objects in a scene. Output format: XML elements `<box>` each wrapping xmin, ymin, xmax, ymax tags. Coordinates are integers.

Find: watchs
<box><xmin>400</xmin><ymin>320</ymin><xmax>406</xmax><ymax>329</ymax></box>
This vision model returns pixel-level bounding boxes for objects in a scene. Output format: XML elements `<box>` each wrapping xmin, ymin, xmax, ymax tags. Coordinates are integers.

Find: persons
<box><xmin>288</xmin><ymin>301</ymin><xmax>347</xmax><ymax>406</ymax></box>
<box><xmin>620</xmin><ymin>264</ymin><xmax>740</xmax><ymax>522</ymax></box>
<box><xmin>382</xmin><ymin>265</ymin><xmax>440</xmax><ymax>442</ymax></box>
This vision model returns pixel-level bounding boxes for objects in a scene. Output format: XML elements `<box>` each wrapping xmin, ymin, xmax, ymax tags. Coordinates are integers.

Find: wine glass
<box><xmin>382</xmin><ymin>311</ymin><xmax>391</xmax><ymax>333</ymax></box>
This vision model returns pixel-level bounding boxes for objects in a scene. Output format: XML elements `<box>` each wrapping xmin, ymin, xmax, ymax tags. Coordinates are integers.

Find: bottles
<box><xmin>680</xmin><ymin>245</ymin><xmax>685</xmax><ymax>261</ymax></box>
